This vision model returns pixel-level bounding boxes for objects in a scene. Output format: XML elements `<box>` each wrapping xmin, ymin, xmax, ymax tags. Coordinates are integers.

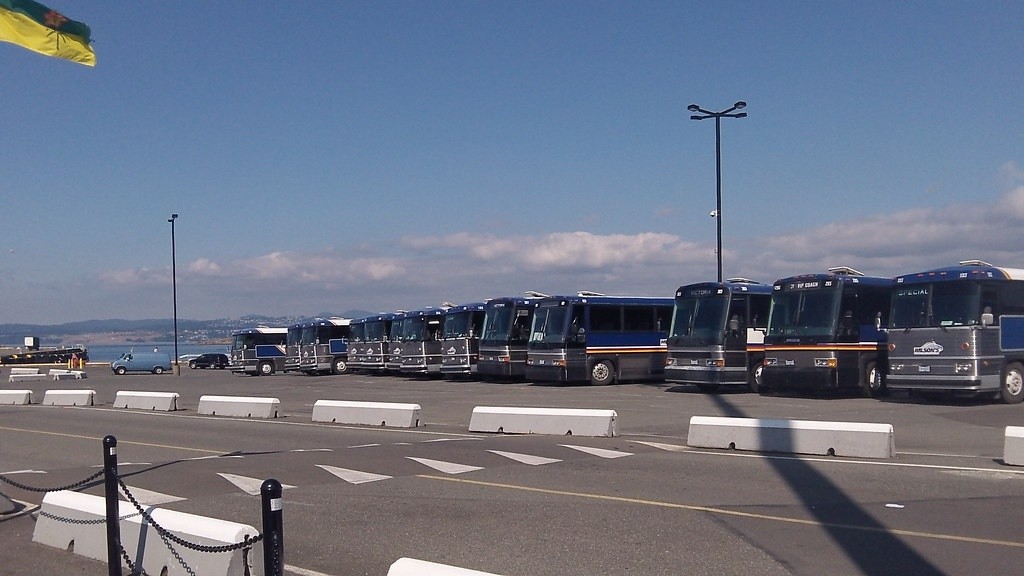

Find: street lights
<box><xmin>687</xmin><ymin>100</ymin><xmax>749</xmax><ymax>283</ymax></box>
<box><xmin>168</xmin><ymin>213</ymin><xmax>179</xmax><ymax>365</ymax></box>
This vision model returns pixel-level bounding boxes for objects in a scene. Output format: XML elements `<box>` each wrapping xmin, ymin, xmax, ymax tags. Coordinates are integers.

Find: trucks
<box><xmin>110</xmin><ymin>352</ymin><xmax>173</xmax><ymax>374</ymax></box>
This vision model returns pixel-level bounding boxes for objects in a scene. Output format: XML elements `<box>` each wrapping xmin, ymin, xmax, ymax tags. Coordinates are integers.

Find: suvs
<box><xmin>189</xmin><ymin>353</ymin><xmax>230</xmax><ymax>370</ymax></box>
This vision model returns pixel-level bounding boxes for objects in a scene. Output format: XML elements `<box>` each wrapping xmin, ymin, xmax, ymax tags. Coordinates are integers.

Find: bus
<box><xmin>526</xmin><ymin>289</ymin><xmax>676</xmax><ymax>387</ymax></box>
<box><xmin>228</xmin><ymin>327</ymin><xmax>288</xmax><ymax>377</ymax></box>
<box><xmin>760</xmin><ymin>265</ymin><xmax>892</xmax><ymax>399</ymax></box>
<box><xmin>475</xmin><ymin>289</ymin><xmax>548</xmax><ymax>380</ymax></box>
<box><xmin>666</xmin><ymin>276</ymin><xmax>774</xmax><ymax>393</ymax></box>
<box><xmin>287</xmin><ymin>306</ymin><xmax>446</xmax><ymax>378</ymax></box>
<box><xmin>884</xmin><ymin>259</ymin><xmax>1024</xmax><ymax>405</ymax></box>
<box><xmin>440</xmin><ymin>302</ymin><xmax>489</xmax><ymax>378</ymax></box>
